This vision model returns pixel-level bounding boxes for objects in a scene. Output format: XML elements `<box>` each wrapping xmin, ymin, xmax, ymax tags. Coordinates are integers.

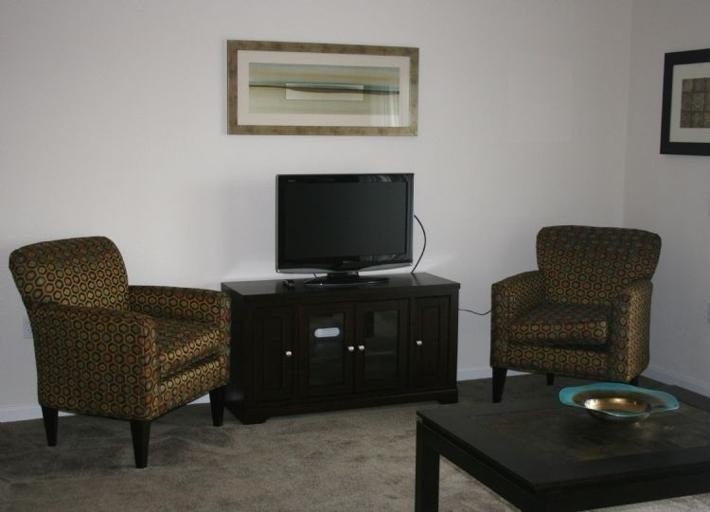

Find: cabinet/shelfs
<box><xmin>220</xmin><ymin>272</ymin><xmax>460</xmax><ymax>424</ymax></box>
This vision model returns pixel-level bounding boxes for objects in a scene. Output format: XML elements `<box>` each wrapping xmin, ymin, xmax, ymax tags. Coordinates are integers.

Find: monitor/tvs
<box><xmin>276</xmin><ymin>173</ymin><xmax>415</xmax><ymax>286</ymax></box>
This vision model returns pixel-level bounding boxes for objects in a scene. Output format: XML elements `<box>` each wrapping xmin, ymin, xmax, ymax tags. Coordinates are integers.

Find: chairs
<box><xmin>490</xmin><ymin>225</ymin><xmax>661</xmax><ymax>405</ymax></box>
<box><xmin>8</xmin><ymin>238</ymin><xmax>235</xmax><ymax>467</ymax></box>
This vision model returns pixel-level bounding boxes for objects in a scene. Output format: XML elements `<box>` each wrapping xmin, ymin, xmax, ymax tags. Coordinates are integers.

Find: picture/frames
<box><xmin>660</xmin><ymin>48</ymin><xmax>710</xmax><ymax>157</ymax></box>
<box><xmin>227</xmin><ymin>40</ymin><xmax>419</xmax><ymax>135</ymax></box>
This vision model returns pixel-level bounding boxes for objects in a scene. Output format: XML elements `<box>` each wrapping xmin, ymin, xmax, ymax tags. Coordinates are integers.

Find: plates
<box><xmin>558</xmin><ymin>381</ymin><xmax>680</xmax><ymax>425</ymax></box>
